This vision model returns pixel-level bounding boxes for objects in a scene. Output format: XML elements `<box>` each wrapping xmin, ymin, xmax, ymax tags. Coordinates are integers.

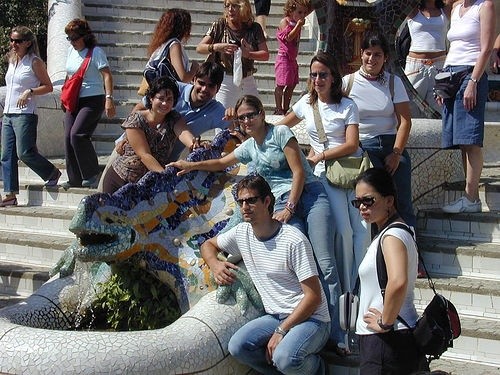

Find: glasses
<box><xmin>66</xmin><ymin>33</ymin><xmax>86</xmax><ymax>41</ymax></box>
<box><xmin>237</xmin><ymin>193</ymin><xmax>268</xmax><ymax>208</ymax></box>
<box><xmin>223</xmin><ymin>5</ymin><xmax>240</xmax><ymax>10</ymax></box>
<box><xmin>309</xmin><ymin>72</ymin><xmax>332</xmax><ymax>78</ymax></box>
<box><xmin>239</xmin><ymin>111</ymin><xmax>260</xmax><ymax>120</ymax></box>
<box><xmin>11</xmin><ymin>38</ymin><xmax>29</xmax><ymax>43</ymax></box>
<box><xmin>351</xmin><ymin>195</ymin><xmax>386</xmax><ymax>208</ymax></box>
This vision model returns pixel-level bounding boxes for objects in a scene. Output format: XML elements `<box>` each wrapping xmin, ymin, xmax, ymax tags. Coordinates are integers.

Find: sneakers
<box><xmin>441</xmin><ymin>196</ymin><xmax>482</xmax><ymax>213</ymax></box>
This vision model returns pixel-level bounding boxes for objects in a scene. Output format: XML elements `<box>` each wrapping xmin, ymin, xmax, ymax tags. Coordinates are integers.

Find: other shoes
<box><xmin>82</xmin><ymin>171</ymin><xmax>102</xmax><ymax>187</ymax></box>
<box><xmin>45</xmin><ymin>170</ymin><xmax>62</xmax><ymax>185</ymax></box>
<box><xmin>273</xmin><ymin>108</ymin><xmax>283</xmax><ymax>115</ymax></box>
<box><xmin>61</xmin><ymin>181</ymin><xmax>82</xmax><ymax>187</ymax></box>
<box><xmin>0</xmin><ymin>198</ymin><xmax>17</xmax><ymax>207</ymax></box>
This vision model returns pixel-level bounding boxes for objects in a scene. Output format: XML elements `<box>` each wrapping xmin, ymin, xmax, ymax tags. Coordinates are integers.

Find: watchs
<box><xmin>377</xmin><ymin>316</ymin><xmax>394</xmax><ymax>330</ymax></box>
<box><xmin>286</xmin><ymin>202</ymin><xmax>296</xmax><ymax>211</ymax></box>
<box><xmin>275</xmin><ymin>327</ymin><xmax>286</xmax><ymax>335</ymax></box>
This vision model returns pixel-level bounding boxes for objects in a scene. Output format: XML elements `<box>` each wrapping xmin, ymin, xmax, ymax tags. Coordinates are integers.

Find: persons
<box><xmin>349</xmin><ymin>167</ymin><xmax>430</xmax><ymax>375</ymax></box>
<box><xmin>339</xmin><ymin>32</ymin><xmax>415</xmax><ymax>230</ymax></box>
<box><xmin>274</xmin><ymin>0</ymin><xmax>313</xmax><ymax>116</ymax></box>
<box><xmin>274</xmin><ymin>51</ymin><xmax>371</xmax><ymax>300</ymax></box>
<box><xmin>60</xmin><ymin>19</ymin><xmax>116</xmax><ymax>189</ymax></box>
<box><xmin>97</xmin><ymin>0</ymin><xmax>271</xmax><ymax>194</ymax></box>
<box><xmin>0</xmin><ymin>25</ymin><xmax>61</xmax><ymax>208</ymax></box>
<box><xmin>166</xmin><ymin>95</ymin><xmax>353</xmax><ymax>356</ymax></box>
<box><xmin>200</xmin><ymin>173</ymin><xmax>330</xmax><ymax>375</ymax></box>
<box><xmin>403</xmin><ymin>0</ymin><xmax>500</xmax><ymax>213</ymax></box>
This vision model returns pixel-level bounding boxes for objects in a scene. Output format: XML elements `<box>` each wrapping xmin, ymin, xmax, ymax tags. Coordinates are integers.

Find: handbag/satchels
<box><xmin>410</xmin><ymin>293</ymin><xmax>461</xmax><ymax>364</ymax></box>
<box><xmin>232</xmin><ymin>47</ymin><xmax>242</xmax><ymax>86</ymax></box>
<box><xmin>325</xmin><ymin>151</ymin><xmax>374</xmax><ymax>189</ymax></box>
<box><xmin>142</xmin><ymin>39</ymin><xmax>183</xmax><ymax>91</ymax></box>
<box><xmin>432</xmin><ymin>72</ymin><xmax>466</xmax><ymax>98</ymax></box>
<box><xmin>60</xmin><ymin>73</ymin><xmax>84</xmax><ymax>113</ymax></box>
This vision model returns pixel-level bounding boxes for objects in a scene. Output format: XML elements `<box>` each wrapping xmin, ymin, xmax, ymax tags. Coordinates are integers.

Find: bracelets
<box><xmin>29</xmin><ymin>88</ymin><xmax>33</xmax><ymax>92</ymax></box>
<box><xmin>106</xmin><ymin>95</ymin><xmax>115</xmax><ymax>101</ymax></box>
<box><xmin>393</xmin><ymin>151</ymin><xmax>400</xmax><ymax>155</ymax></box>
<box><xmin>285</xmin><ymin>207</ymin><xmax>292</xmax><ymax>214</ymax></box>
<box><xmin>470</xmin><ymin>78</ymin><xmax>478</xmax><ymax>83</ymax></box>
<box><xmin>322</xmin><ymin>152</ymin><xmax>325</xmax><ymax>160</ymax></box>
<box><xmin>208</xmin><ymin>44</ymin><xmax>213</xmax><ymax>52</ymax></box>
